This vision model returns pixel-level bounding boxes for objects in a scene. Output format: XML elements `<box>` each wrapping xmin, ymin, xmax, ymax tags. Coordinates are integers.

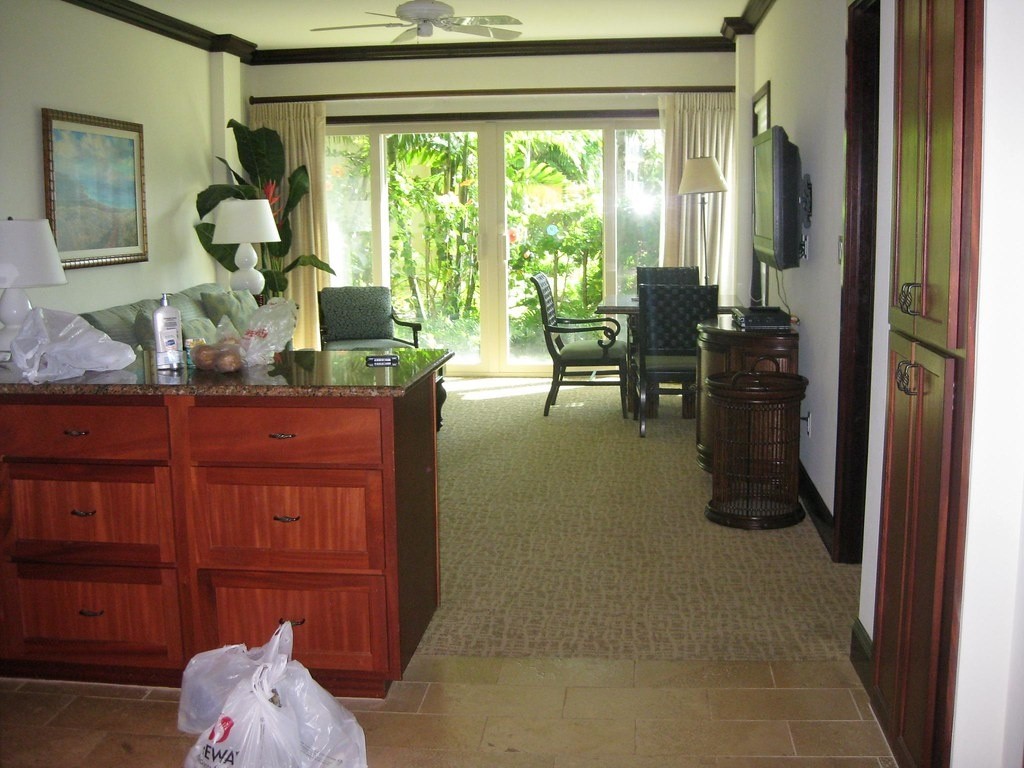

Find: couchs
<box><xmin>78</xmin><ymin>283</ymin><xmax>231</xmax><ymax>362</ymax></box>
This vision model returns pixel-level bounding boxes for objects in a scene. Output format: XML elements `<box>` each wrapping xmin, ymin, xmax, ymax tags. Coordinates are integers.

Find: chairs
<box><xmin>634</xmin><ymin>267</ymin><xmax>719</xmax><ymax>432</ymax></box>
<box><xmin>531</xmin><ymin>272</ymin><xmax>633</xmax><ymax>420</ymax></box>
<box><xmin>317</xmin><ymin>287</ymin><xmax>447</xmax><ymax>431</ymax></box>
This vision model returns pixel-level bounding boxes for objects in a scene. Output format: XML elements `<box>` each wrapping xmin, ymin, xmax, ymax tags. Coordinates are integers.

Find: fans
<box><xmin>310</xmin><ymin>2</ymin><xmax>524</xmax><ymax>48</ymax></box>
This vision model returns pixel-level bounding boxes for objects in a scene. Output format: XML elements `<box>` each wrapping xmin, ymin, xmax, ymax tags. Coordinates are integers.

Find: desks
<box><xmin>594</xmin><ymin>304</ymin><xmax>746</xmax><ymax>416</ymax></box>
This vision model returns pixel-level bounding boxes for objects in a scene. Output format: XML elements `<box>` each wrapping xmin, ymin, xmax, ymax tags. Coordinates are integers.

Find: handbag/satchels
<box><xmin>177</xmin><ymin>620</ymin><xmax>294</xmax><ymax>734</ymax></box>
<box><xmin>185</xmin><ymin>653</ymin><xmax>366</xmax><ymax>768</ymax></box>
<box><xmin>10</xmin><ymin>307</ymin><xmax>136</xmax><ymax>386</ymax></box>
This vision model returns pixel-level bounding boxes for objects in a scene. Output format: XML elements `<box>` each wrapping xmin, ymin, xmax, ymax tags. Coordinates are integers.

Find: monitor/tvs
<box><xmin>751</xmin><ymin>124</ymin><xmax>803</xmax><ymax>271</ymax></box>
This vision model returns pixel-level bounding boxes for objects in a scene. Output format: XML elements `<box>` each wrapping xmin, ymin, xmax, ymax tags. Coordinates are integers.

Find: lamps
<box><xmin>0</xmin><ymin>219</ymin><xmax>69</xmax><ymax>350</ymax></box>
<box><xmin>211</xmin><ymin>199</ymin><xmax>281</xmax><ymax>294</ymax></box>
<box><xmin>678</xmin><ymin>156</ymin><xmax>730</xmax><ymax>284</ymax></box>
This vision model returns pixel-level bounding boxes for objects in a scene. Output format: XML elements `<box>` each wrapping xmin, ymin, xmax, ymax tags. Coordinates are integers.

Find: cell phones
<box><xmin>366</xmin><ymin>355</ymin><xmax>398</xmax><ymax>363</ymax></box>
<box><xmin>367</xmin><ymin>362</ymin><xmax>398</xmax><ymax>367</ymax></box>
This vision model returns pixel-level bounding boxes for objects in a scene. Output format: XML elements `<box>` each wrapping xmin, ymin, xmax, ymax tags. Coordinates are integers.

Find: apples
<box><xmin>195</xmin><ymin>338</ymin><xmax>242</xmax><ymax>371</ymax></box>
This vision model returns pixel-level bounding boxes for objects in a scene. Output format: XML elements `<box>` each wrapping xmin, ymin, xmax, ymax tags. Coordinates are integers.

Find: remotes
<box><xmin>747</xmin><ymin>306</ymin><xmax>780</xmax><ymax>312</ymax></box>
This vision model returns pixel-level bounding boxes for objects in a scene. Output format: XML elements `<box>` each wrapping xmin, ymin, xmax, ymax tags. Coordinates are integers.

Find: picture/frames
<box><xmin>41</xmin><ymin>107</ymin><xmax>149</xmax><ymax>270</ymax></box>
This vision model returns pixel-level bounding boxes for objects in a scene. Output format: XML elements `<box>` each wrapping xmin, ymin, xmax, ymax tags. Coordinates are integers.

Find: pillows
<box><xmin>136</xmin><ymin>307</ymin><xmax>158</xmax><ymax>350</ymax></box>
<box><xmin>200</xmin><ymin>289</ymin><xmax>260</xmax><ymax>332</ymax></box>
<box><xmin>181</xmin><ymin>315</ymin><xmax>218</xmax><ymax>348</ymax></box>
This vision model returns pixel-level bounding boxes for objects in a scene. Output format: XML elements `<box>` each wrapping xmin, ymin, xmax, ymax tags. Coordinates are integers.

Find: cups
<box><xmin>185</xmin><ymin>338</ymin><xmax>206</xmax><ymax>364</ymax></box>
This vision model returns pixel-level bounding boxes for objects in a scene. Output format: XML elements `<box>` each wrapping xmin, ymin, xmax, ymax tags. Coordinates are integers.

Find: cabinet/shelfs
<box><xmin>0</xmin><ymin>372</ymin><xmax>439</xmax><ymax>698</ymax></box>
<box><xmin>697</xmin><ymin>319</ymin><xmax>799</xmax><ymax>472</ymax></box>
<box><xmin>870</xmin><ymin>0</ymin><xmax>985</xmax><ymax>768</ymax></box>
